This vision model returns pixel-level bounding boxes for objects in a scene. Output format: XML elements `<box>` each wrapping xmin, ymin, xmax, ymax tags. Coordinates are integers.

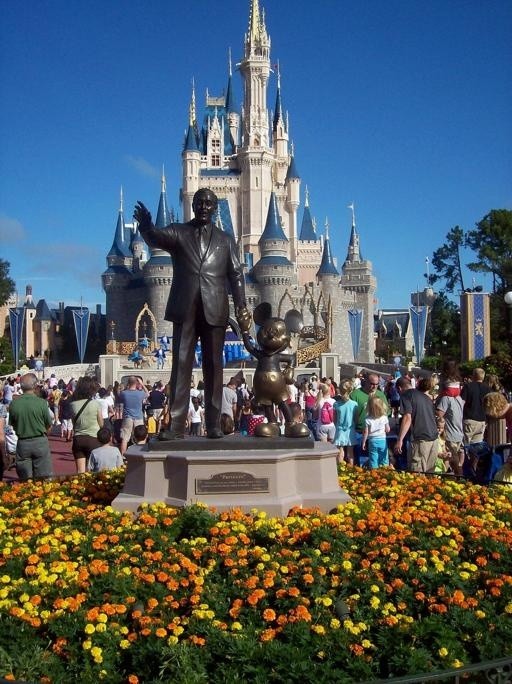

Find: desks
<box><xmin>425</xmin><ymin>256</ymin><xmax>430</xmax><ymax>288</ymax></box>
<box><xmin>35</xmin><ymin>360</ymin><xmax>43</xmax><ymax>380</ymax></box>
<box><xmin>394</xmin><ymin>356</ymin><xmax>400</xmax><ymax>369</ymax></box>
<box><xmin>504</xmin><ymin>291</ymin><xmax>512</xmax><ymax>307</ymax></box>
<box><xmin>107</xmin><ymin>319</ymin><xmax>117</xmax><ymax>354</ymax></box>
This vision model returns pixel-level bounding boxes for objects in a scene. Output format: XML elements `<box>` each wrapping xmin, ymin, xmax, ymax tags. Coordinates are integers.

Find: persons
<box><xmin>0</xmin><ymin>363</ymin><xmax>512</xmax><ymax>476</ymax></box>
<box><xmin>192</xmin><ymin>336</ymin><xmax>202</xmax><ymax>367</ymax></box>
<box><xmin>132</xmin><ymin>185</ymin><xmax>250</xmax><ymax>441</ymax></box>
<box><xmin>223</xmin><ymin>341</ymin><xmax>249</xmax><ymax>367</ymax></box>
<box><xmin>128</xmin><ymin>332</ymin><xmax>171</xmax><ymax>369</ymax></box>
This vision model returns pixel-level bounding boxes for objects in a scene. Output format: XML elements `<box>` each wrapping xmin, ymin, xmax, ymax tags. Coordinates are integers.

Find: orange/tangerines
<box><xmin>461</xmin><ymin>438</ymin><xmax>512</xmax><ymax>485</ymax></box>
<box><xmin>109</xmin><ymin>414</ymin><xmax>136</xmax><ymax>446</ymax></box>
<box><xmin>361</xmin><ymin>433</ymin><xmax>409</xmax><ymax>471</ymax></box>
